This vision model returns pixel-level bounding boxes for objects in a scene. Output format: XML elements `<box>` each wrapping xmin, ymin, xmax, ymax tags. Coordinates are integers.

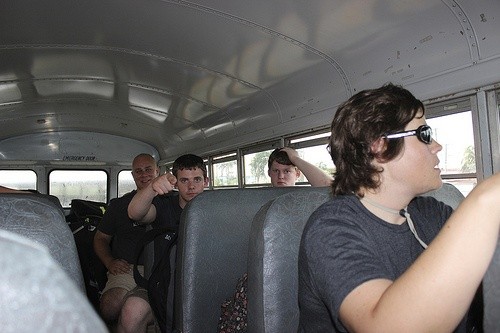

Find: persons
<box><xmin>268</xmin><ymin>147</ymin><xmax>335</xmax><ymax>188</ymax></box>
<box><xmin>0</xmin><ymin>186</ymin><xmax>42</xmax><ymax>194</ymax></box>
<box><xmin>295</xmin><ymin>83</ymin><xmax>500</xmax><ymax>333</ymax></box>
<box><xmin>93</xmin><ymin>153</ymin><xmax>161</xmax><ymax>333</ymax></box>
<box><xmin>116</xmin><ymin>154</ymin><xmax>209</xmax><ymax>333</ymax></box>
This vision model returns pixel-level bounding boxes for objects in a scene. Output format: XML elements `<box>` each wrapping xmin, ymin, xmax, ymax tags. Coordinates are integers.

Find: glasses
<box><xmin>380</xmin><ymin>125</ymin><xmax>434</xmax><ymax>145</ymax></box>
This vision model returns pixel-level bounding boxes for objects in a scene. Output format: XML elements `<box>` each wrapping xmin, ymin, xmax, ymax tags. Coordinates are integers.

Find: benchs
<box><xmin>0</xmin><ymin>189</ymin><xmax>110</xmax><ymax>333</ymax></box>
<box><xmin>143</xmin><ymin>183</ymin><xmax>500</xmax><ymax>333</ymax></box>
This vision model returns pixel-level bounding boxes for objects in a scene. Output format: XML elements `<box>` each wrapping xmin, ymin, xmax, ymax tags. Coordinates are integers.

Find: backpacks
<box><xmin>216</xmin><ymin>271</ymin><xmax>248</xmax><ymax>333</ymax></box>
